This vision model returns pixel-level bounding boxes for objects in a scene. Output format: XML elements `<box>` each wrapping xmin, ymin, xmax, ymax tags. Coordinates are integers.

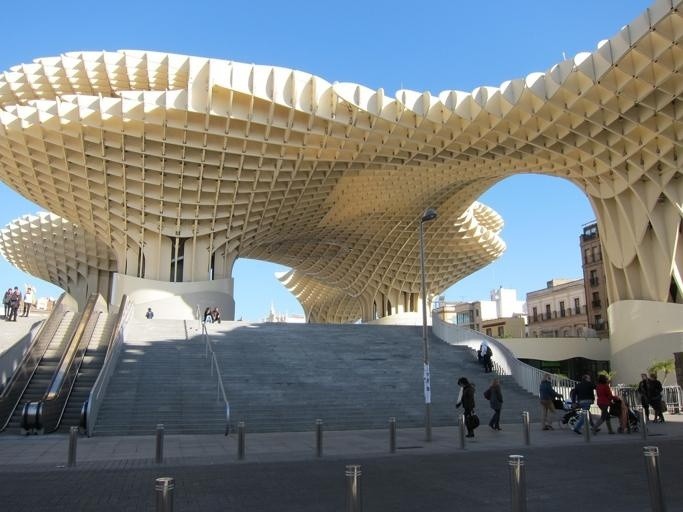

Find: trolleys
<box><xmin>553</xmin><ymin>394</ymin><xmax>585</xmax><ymax>430</ymax></box>
<box><xmin>609</xmin><ymin>396</ymin><xmax>641</xmax><ymax>432</ymax></box>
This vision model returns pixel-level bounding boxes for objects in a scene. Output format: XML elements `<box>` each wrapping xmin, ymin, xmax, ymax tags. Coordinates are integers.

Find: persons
<box><xmin>146</xmin><ymin>307</ymin><xmax>153</xmax><ymax>319</ymax></box>
<box><xmin>20</xmin><ymin>287</ymin><xmax>33</xmax><ymax>317</ymax></box>
<box><xmin>480</xmin><ymin>342</ymin><xmax>494</xmax><ymax>373</ymax></box>
<box><xmin>571</xmin><ymin>373</ymin><xmax>600</xmax><ymax>435</ymax></box>
<box><xmin>458</xmin><ymin>377</ymin><xmax>475</xmax><ymax>438</ymax></box>
<box><xmin>540</xmin><ymin>372</ymin><xmax>563</xmax><ymax>431</ymax></box>
<box><xmin>3</xmin><ymin>286</ymin><xmax>22</xmax><ymax>322</ymax></box>
<box><xmin>638</xmin><ymin>371</ymin><xmax>665</xmax><ymax>424</ymax></box>
<box><xmin>203</xmin><ymin>307</ymin><xmax>221</xmax><ymax>324</ymax></box>
<box><xmin>592</xmin><ymin>372</ymin><xmax>620</xmax><ymax>434</ymax></box>
<box><xmin>488</xmin><ymin>378</ymin><xmax>504</xmax><ymax>430</ymax></box>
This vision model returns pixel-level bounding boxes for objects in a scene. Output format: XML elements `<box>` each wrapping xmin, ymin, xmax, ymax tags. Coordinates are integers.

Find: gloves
<box><xmin>456</xmin><ymin>403</ymin><xmax>462</xmax><ymax>408</ymax></box>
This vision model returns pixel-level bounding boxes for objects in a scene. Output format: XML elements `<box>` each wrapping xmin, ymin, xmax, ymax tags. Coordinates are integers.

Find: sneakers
<box><xmin>542</xmin><ymin>425</ymin><xmax>552</xmax><ymax>430</ymax></box>
<box><xmin>573</xmin><ymin>429</ymin><xmax>581</xmax><ymax>434</ymax></box>
<box><xmin>466</xmin><ymin>434</ymin><xmax>475</xmax><ymax>437</ymax></box>
<box><xmin>653</xmin><ymin>418</ymin><xmax>665</xmax><ymax>424</ymax></box>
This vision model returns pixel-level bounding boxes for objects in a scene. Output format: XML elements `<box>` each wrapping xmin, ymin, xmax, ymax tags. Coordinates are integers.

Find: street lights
<box><xmin>418</xmin><ymin>209</ymin><xmax>437</xmax><ymax>444</ymax></box>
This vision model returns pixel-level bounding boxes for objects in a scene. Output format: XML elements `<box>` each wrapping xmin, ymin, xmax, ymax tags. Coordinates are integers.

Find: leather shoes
<box><xmin>490</xmin><ymin>424</ymin><xmax>502</xmax><ymax>430</ymax></box>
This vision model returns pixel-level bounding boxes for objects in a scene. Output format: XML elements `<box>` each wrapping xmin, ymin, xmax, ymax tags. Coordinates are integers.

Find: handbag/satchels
<box><xmin>484</xmin><ymin>390</ymin><xmax>491</xmax><ymax>400</ymax></box>
<box><xmin>609</xmin><ymin>404</ymin><xmax>620</xmax><ymax>416</ymax></box>
<box><xmin>553</xmin><ymin>400</ymin><xmax>564</xmax><ymax>409</ymax></box>
<box><xmin>466</xmin><ymin>415</ymin><xmax>479</xmax><ymax>429</ymax></box>
<box><xmin>660</xmin><ymin>401</ymin><xmax>667</xmax><ymax>413</ymax></box>
